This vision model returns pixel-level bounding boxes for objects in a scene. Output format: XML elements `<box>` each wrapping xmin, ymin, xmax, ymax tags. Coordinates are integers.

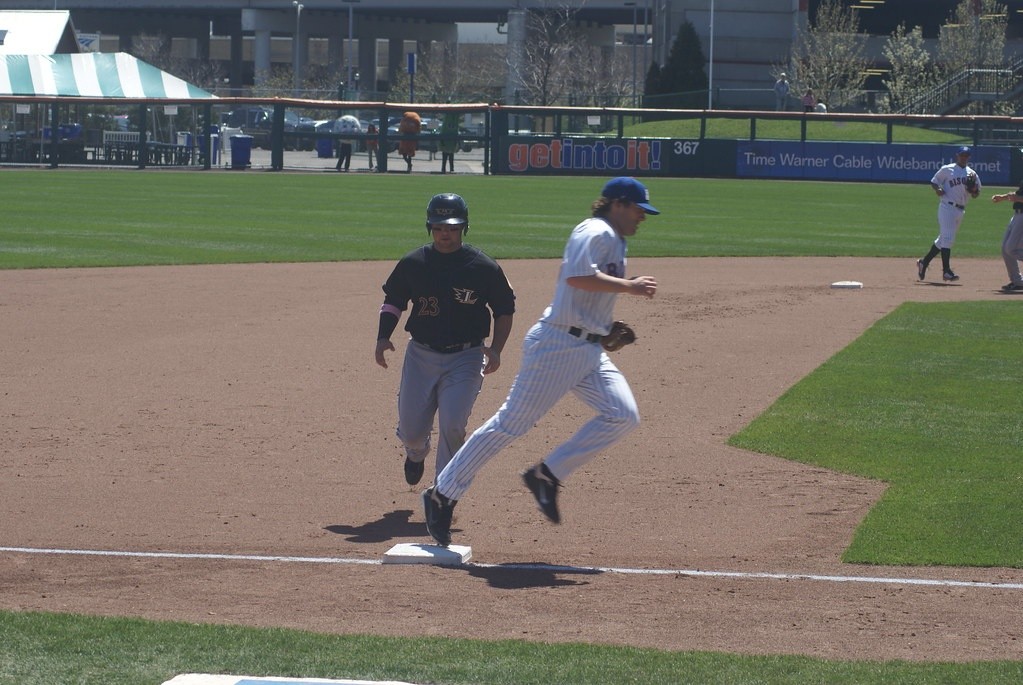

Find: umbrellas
<box><xmin>338</xmin><ymin>115</ymin><xmax>361</xmax><ymax>130</ymax></box>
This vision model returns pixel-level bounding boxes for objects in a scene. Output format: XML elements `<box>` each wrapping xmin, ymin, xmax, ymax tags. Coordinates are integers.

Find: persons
<box><xmin>421</xmin><ymin>177</ymin><xmax>660</xmax><ymax>547</ymax></box>
<box><xmin>375</xmin><ymin>192</ymin><xmax>516</xmax><ymax>485</ymax></box>
<box><xmin>439</xmin><ymin>100</ymin><xmax>458</xmax><ymax>173</ymax></box>
<box><xmin>366</xmin><ymin>124</ymin><xmax>379</xmax><ymax>169</ymax></box>
<box><xmin>803</xmin><ymin>88</ymin><xmax>827</xmax><ymax>112</ymax></box>
<box><xmin>875</xmin><ymin>93</ymin><xmax>908</xmax><ymax>126</ymax></box>
<box><xmin>336</xmin><ymin>120</ymin><xmax>358</xmax><ymax>172</ymax></box>
<box><xmin>774</xmin><ymin>72</ymin><xmax>790</xmax><ymax>111</ymax></box>
<box><xmin>992</xmin><ymin>181</ymin><xmax>1023</xmax><ymax>292</ymax></box>
<box><xmin>917</xmin><ymin>146</ymin><xmax>981</xmax><ymax>281</ymax></box>
<box><xmin>399</xmin><ymin>110</ymin><xmax>421</xmax><ymax>174</ymax></box>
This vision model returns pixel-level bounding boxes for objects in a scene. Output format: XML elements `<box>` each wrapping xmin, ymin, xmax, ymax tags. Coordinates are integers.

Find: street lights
<box><xmin>295</xmin><ymin>3</ymin><xmax>304</xmax><ymax>97</ymax></box>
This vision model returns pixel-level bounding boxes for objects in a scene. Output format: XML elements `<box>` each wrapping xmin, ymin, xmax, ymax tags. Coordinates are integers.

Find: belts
<box><xmin>568</xmin><ymin>326</ymin><xmax>604</xmax><ymax>343</ymax></box>
<box><xmin>949</xmin><ymin>202</ymin><xmax>964</xmax><ymax>209</ymax></box>
<box><xmin>414</xmin><ymin>338</ymin><xmax>481</xmax><ymax>353</ymax></box>
<box><xmin>1015</xmin><ymin>209</ymin><xmax>1023</xmax><ymax>214</ymax></box>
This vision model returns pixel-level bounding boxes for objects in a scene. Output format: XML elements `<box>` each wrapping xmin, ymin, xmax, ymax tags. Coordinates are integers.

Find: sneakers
<box><xmin>916</xmin><ymin>258</ymin><xmax>928</xmax><ymax>280</ymax></box>
<box><xmin>421</xmin><ymin>484</ymin><xmax>458</xmax><ymax>547</ymax></box>
<box><xmin>1001</xmin><ymin>282</ymin><xmax>1023</xmax><ymax>290</ymax></box>
<box><xmin>522</xmin><ymin>464</ymin><xmax>562</xmax><ymax>523</ymax></box>
<box><xmin>943</xmin><ymin>270</ymin><xmax>960</xmax><ymax>280</ymax></box>
<box><xmin>404</xmin><ymin>457</ymin><xmax>424</xmax><ymax>485</ymax></box>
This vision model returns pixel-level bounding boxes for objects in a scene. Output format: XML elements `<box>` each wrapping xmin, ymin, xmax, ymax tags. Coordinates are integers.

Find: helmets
<box><xmin>426</xmin><ymin>193</ymin><xmax>469</xmax><ymax>236</ymax></box>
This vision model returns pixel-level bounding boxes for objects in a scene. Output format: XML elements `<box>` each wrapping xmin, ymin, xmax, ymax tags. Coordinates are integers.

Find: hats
<box><xmin>957</xmin><ymin>146</ymin><xmax>973</xmax><ymax>155</ymax></box>
<box><xmin>603</xmin><ymin>177</ymin><xmax>660</xmax><ymax>215</ymax></box>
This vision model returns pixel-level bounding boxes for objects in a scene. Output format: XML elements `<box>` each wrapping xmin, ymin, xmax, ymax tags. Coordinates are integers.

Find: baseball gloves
<box><xmin>603</xmin><ymin>323</ymin><xmax>637</xmax><ymax>353</ymax></box>
<box><xmin>966</xmin><ymin>172</ymin><xmax>978</xmax><ymax>193</ymax></box>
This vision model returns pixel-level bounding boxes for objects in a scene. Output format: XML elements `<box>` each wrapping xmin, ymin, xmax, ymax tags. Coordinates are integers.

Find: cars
<box><xmin>212</xmin><ymin>107</ymin><xmax>479</xmax><ymax>155</ymax></box>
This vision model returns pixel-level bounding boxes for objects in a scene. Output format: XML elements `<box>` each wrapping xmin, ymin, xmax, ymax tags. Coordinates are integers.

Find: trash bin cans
<box><xmin>229</xmin><ymin>133</ymin><xmax>254</xmax><ymax>169</ymax></box>
<box><xmin>318</xmin><ymin>128</ymin><xmax>333</xmax><ymax>158</ymax></box>
<box><xmin>197</xmin><ymin>134</ymin><xmax>218</xmax><ymax>165</ymax></box>
<box><xmin>60</xmin><ymin>123</ymin><xmax>82</xmax><ymax>136</ymax></box>
<box><xmin>43</xmin><ymin>125</ymin><xmax>53</xmax><ymax>139</ymax></box>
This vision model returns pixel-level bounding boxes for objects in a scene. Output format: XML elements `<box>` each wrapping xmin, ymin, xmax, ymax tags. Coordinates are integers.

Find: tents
<box><xmin>0</xmin><ymin>52</ymin><xmax>220</xmax><ymax>99</ymax></box>
